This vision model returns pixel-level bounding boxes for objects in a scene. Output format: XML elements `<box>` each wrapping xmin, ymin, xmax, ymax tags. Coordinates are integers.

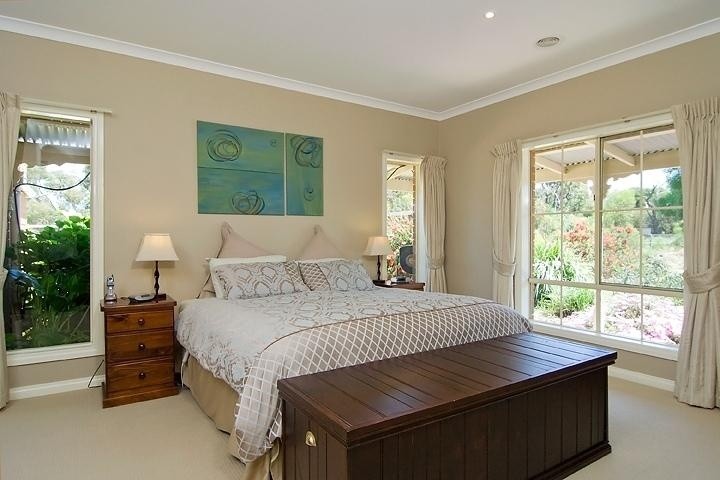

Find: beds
<box><xmin>175</xmin><ymin>285</ymin><xmax>533</xmax><ymax>480</ymax></box>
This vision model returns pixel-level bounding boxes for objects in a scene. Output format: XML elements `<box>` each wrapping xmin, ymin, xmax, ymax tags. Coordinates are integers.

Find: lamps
<box><xmin>363</xmin><ymin>236</ymin><xmax>394</xmax><ymax>283</ymax></box>
<box><xmin>135</xmin><ymin>231</ymin><xmax>180</xmax><ymax>301</ymax></box>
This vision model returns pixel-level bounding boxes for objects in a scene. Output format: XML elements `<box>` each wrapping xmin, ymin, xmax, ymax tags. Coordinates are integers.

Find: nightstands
<box><xmin>376</xmin><ymin>282</ymin><xmax>425</xmax><ymax>291</ymax></box>
<box><xmin>100</xmin><ymin>293</ymin><xmax>179</xmax><ymax>408</ymax></box>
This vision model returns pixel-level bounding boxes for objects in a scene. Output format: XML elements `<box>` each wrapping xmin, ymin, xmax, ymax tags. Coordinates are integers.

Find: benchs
<box><xmin>277</xmin><ymin>331</ymin><xmax>618</xmax><ymax>478</ymax></box>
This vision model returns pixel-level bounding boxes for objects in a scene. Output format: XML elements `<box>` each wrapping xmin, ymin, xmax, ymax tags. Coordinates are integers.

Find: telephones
<box><xmin>105</xmin><ymin>274</ymin><xmax>117</xmax><ymax>301</ymax></box>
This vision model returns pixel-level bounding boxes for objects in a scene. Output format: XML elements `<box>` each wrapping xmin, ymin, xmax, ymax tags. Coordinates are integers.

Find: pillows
<box><xmin>198</xmin><ymin>224</ymin><xmax>375</xmax><ymax>299</ymax></box>
